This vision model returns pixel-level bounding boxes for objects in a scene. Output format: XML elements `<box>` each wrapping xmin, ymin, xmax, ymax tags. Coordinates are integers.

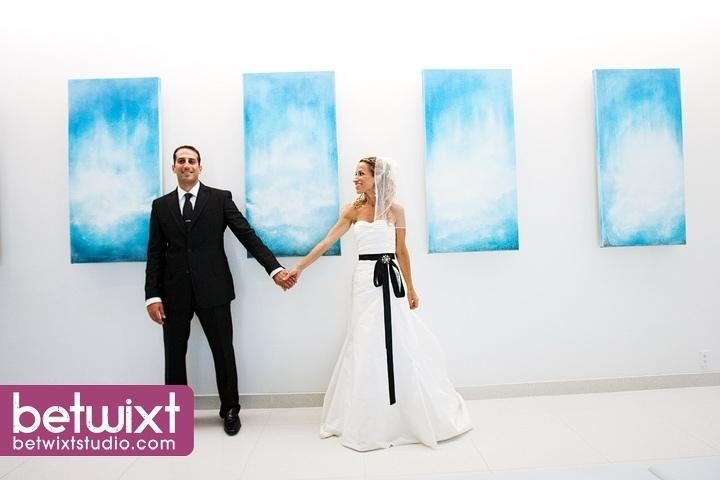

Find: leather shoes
<box><xmin>217</xmin><ymin>407</ymin><xmax>242</xmax><ymax>436</ymax></box>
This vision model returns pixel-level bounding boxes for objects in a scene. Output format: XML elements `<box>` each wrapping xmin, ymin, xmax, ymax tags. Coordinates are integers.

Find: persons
<box><xmin>145</xmin><ymin>146</ymin><xmax>297</xmax><ymax>436</ymax></box>
<box><xmin>283</xmin><ymin>155</ymin><xmax>475</xmax><ymax>452</ymax></box>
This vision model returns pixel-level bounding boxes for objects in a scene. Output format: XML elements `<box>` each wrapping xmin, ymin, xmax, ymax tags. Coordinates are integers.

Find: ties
<box><xmin>180</xmin><ymin>193</ymin><xmax>195</xmax><ymax>220</ymax></box>
